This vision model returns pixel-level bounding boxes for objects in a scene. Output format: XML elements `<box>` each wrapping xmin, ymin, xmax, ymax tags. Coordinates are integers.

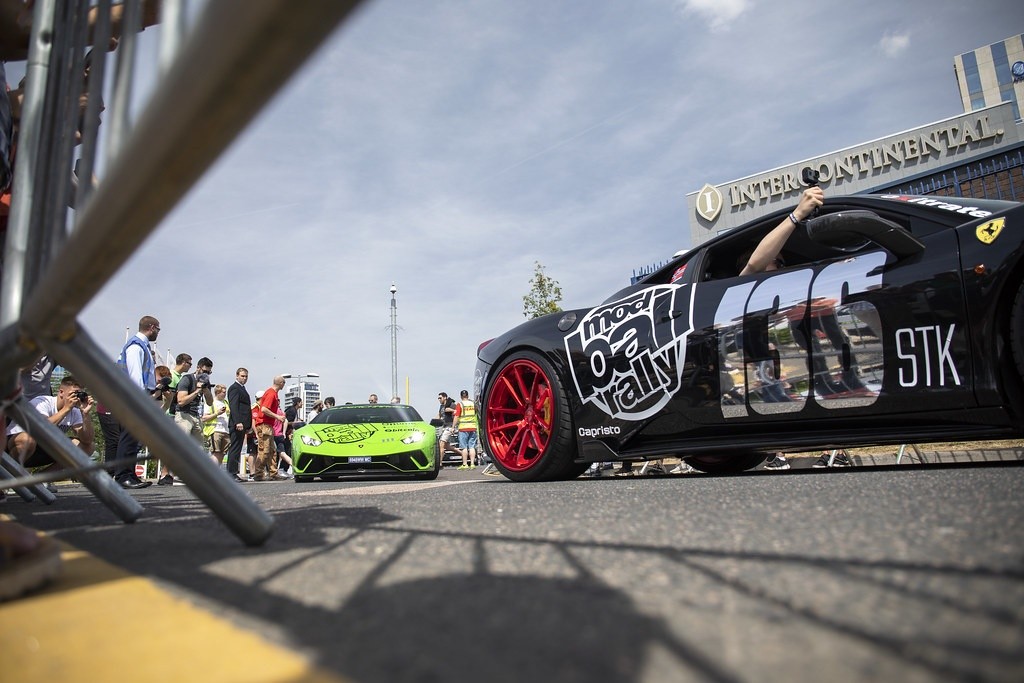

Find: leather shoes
<box><xmin>120</xmin><ymin>477</ymin><xmax>153</xmax><ymax>489</ymax></box>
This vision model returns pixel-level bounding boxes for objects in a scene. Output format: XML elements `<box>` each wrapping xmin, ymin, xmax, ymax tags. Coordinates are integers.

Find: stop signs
<box><xmin>135</xmin><ymin>465</ymin><xmax>146</xmax><ymax>477</ymax></box>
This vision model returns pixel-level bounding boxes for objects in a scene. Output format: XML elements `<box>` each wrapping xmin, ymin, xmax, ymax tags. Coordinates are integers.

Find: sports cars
<box><xmin>291</xmin><ymin>402</ymin><xmax>442</xmax><ymax>483</ymax></box>
<box><xmin>474</xmin><ymin>195</ymin><xmax>1024</xmax><ymax>482</ymax></box>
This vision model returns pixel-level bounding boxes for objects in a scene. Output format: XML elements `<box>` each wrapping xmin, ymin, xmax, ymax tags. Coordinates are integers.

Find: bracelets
<box><xmin>789</xmin><ymin>213</ymin><xmax>799</xmax><ymax>226</ymax></box>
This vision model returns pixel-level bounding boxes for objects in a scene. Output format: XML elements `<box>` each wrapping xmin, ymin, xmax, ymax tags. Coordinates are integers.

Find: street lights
<box><xmin>384</xmin><ymin>282</ymin><xmax>405</xmax><ymax>403</ymax></box>
<box><xmin>281</xmin><ymin>373</ymin><xmax>320</xmax><ymax>420</ymax></box>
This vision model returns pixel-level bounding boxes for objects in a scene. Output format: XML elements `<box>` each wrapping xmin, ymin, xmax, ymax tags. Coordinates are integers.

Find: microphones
<box><xmin>160</xmin><ymin>377</ymin><xmax>172</xmax><ymax>385</ymax></box>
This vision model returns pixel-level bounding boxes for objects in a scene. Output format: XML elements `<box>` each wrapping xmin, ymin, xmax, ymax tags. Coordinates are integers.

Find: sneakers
<box><xmin>811</xmin><ymin>450</ymin><xmax>852</xmax><ymax>468</ymax></box>
<box><xmin>457</xmin><ymin>465</ymin><xmax>476</xmax><ymax>470</ymax></box>
<box><xmin>578</xmin><ymin>463</ymin><xmax>703</xmax><ymax>478</ymax></box>
<box><xmin>764</xmin><ymin>458</ymin><xmax>790</xmax><ymax>470</ymax></box>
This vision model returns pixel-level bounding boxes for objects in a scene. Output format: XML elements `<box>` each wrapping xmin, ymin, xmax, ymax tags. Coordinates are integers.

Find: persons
<box><xmin>438</xmin><ymin>392</ymin><xmax>462</xmax><ymax>467</ymax></box>
<box><xmin>227</xmin><ymin>368</ymin><xmax>252</xmax><ymax>483</ymax></box>
<box><xmin>247</xmin><ymin>376</ymin><xmax>303</xmax><ymax>481</ymax></box>
<box><xmin>203</xmin><ymin>386</ymin><xmax>230</xmax><ymax>465</ymax></box>
<box><xmin>754</xmin><ymin>344</ymin><xmax>792</xmax><ymax>402</ymax></box>
<box><xmin>117</xmin><ymin>316</ymin><xmax>160</xmax><ymax>488</ymax></box>
<box><xmin>452</xmin><ymin>390</ymin><xmax>478</xmax><ymax>470</ymax></box>
<box><xmin>369</xmin><ymin>394</ymin><xmax>378</xmax><ymax>404</ymax></box>
<box><xmin>391</xmin><ymin>397</ymin><xmax>400</xmax><ymax>403</ymax></box>
<box><xmin>157</xmin><ymin>354</ymin><xmax>192</xmax><ymax>415</ymax></box>
<box><xmin>307</xmin><ymin>400</ymin><xmax>324</xmax><ymax>423</ymax></box>
<box><xmin>580</xmin><ymin>461</ymin><xmax>693</xmax><ymax>476</ymax></box>
<box><xmin>324</xmin><ymin>397</ymin><xmax>335</xmax><ymax>408</ymax></box>
<box><xmin>0</xmin><ymin>0</ymin><xmax>163</xmax><ymax>261</ymax></box>
<box><xmin>739</xmin><ymin>185</ymin><xmax>824</xmax><ymax>274</ymax></box>
<box><xmin>788</xmin><ymin>297</ymin><xmax>880</xmax><ymax>400</ymax></box>
<box><xmin>23</xmin><ymin>357</ymin><xmax>59</xmax><ymax>492</ymax></box>
<box><xmin>95</xmin><ymin>366</ymin><xmax>172</xmax><ymax>478</ymax></box>
<box><xmin>157</xmin><ymin>358</ymin><xmax>213</xmax><ymax>486</ymax></box>
<box><xmin>5</xmin><ymin>378</ymin><xmax>95</xmax><ymax>475</ymax></box>
<box><xmin>763</xmin><ymin>449</ymin><xmax>851</xmax><ymax>469</ymax></box>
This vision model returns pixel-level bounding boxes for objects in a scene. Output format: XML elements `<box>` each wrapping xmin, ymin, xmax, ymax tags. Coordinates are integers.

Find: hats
<box><xmin>312</xmin><ymin>400</ymin><xmax>322</xmax><ymax>409</ymax></box>
<box><xmin>255</xmin><ymin>391</ymin><xmax>265</xmax><ymax>399</ymax></box>
<box><xmin>461</xmin><ymin>390</ymin><xmax>468</xmax><ymax>397</ymax></box>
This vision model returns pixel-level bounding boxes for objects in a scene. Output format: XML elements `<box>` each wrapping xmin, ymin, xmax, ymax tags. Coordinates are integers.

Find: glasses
<box><xmin>772</xmin><ymin>258</ymin><xmax>785</xmax><ymax>269</ymax></box>
<box><xmin>200</xmin><ymin>366</ymin><xmax>212</xmax><ymax>375</ymax></box>
<box><xmin>280</xmin><ymin>381</ymin><xmax>286</xmax><ymax>385</ymax></box>
<box><xmin>149</xmin><ymin>324</ymin><xmax>160</xmax><ymax>332</ymax></box>
<box><xmin>438</xmin><ymin>397</ymin><xmax>443</xmax><ymax>400</ymax></box>
<box><xmin>368</xmin><ymin>400</ymin><xmax>373</xmax><ymax>403</ymax></box>
<box><xmin>186</xmin><ymin>361</ymin><xmax>192</xmax><ymax>368</ymax></box>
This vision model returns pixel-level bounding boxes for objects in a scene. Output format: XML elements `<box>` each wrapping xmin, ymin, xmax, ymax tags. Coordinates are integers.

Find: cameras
<box><xmin>72</xmin><ymin>392</ymin><xmax>88</xmax><ymax>402</ymax></box>
<box><xmin>202</xmin><ymin>382</ymin><xmax>216</xmax><ymax>389</ymax></box>
<box><xmin>802</xmin><ymin>167</ymin><xmax>820</xmax><ymax>184</ymax></box>
<box><xmin>161</xmin><ymin>384</ymin><xmax>178</xmax><ymax>393</ymax></box>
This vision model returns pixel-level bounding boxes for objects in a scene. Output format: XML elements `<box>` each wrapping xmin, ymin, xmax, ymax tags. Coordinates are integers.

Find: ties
<box><xmin>147</xmin><ymin>343</ymin><xmax>151</xmax><ymax>352</ymax></box>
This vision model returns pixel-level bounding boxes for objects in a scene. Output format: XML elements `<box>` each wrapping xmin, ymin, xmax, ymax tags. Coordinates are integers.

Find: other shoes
<box><xmin>42</xmin><ymin>482</ymin><xmax>57</xmax><ymax>493</ymax></box>
<box><xmin>248</xmin><ymin>473</ymin><xmax>254</xmax><ymax>482</ymax></box>
<box><xmin>233</xmin><ymin>475</ymin><xmax>248</xmax><ymax>482</ymax></box>
<box><xmin>157</xmin><ymin>475</ymin><xmax>173</xmax><ymax>486</ymax></box>
<box><xmin>822</xmin><ymin>387</ymin><xmax>881</xmax><ymax>400</ymax></box>
<box><xmin>255</xmin><ymin>477</ymin><xmax>275</xmax><ymax>481</ymax></box>
<box><xmin>272</xmin><ymin>474</ymin><xmax>287</xmax><ymax>481</ymax></box>
